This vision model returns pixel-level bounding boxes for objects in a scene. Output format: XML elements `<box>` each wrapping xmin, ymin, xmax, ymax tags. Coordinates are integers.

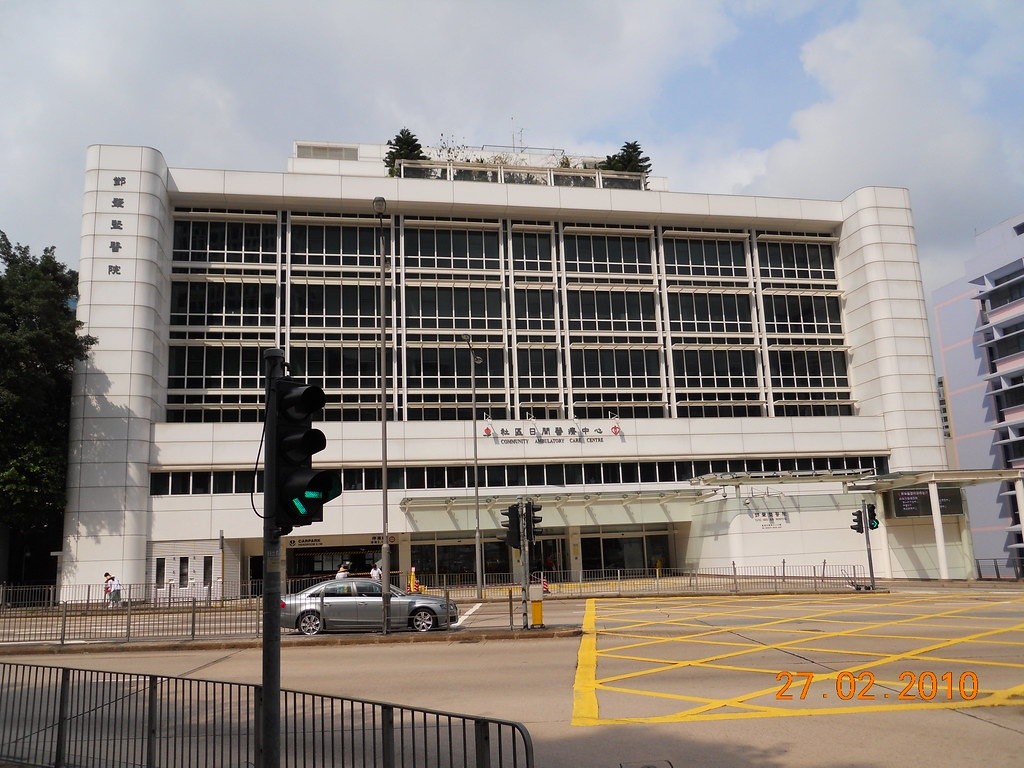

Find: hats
<box><xmin>105</xmin><ymin>575</ymin><xmax>114</xmax><ymax>583</ymax></box>
<box><xmin>338</xmin><ymin>567</ymin><xmax>348</xmax><ymax>572</ymax></box>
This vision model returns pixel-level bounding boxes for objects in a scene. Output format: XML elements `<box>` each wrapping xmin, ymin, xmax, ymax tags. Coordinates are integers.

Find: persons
<box><xmin>371</xmin><ymin>564</ymin><xmax>383</xmax><ymax>596</ymax></box>
<box><xmin>104</xmin><ymin>572</ymin><xmax>121</xmax><ymax>608</ymax></box>
<box><xmin>336</xmin><ymin>567</ymin><xmax>349</xmax><ymax>593</ymax></box>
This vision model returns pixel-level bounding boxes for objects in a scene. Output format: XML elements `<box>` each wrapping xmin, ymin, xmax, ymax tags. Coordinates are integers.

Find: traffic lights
<box><xmin>526</xmin><ymin>498</ymin><xmax>543</xmax><ymax>542</ymax></box>
<box><xmin>867</xmin><ymin>503</ymin><xmax>879</xmax><ymax>530</ymax></box>
<box><xmin>850</xmin><ymin>509</ymin><xmax>863</xmax><ymax>535</ymax></box>
<box><xmin>496</xmin><ymin>504</ymin><xmax>521</xmax><ymax>549</ymax></box>
<box><xmin>275</xmin><ymin>379</ymin><xmax>342</xmax><ymax>533</ymax></box>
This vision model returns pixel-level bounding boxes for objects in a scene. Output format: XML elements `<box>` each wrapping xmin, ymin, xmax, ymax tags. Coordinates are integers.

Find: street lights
<box><xmin>371</xmin><ymin>195</ymin><xmax>389</xmax><ymax>638</ymax></box>
<box><xmin>460</xmin><ymin>332</ymin><xmax>482</xmax><ymax>602</ymax></box>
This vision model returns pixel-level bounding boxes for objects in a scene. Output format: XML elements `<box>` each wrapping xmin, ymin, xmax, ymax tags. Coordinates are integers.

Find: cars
<box><xmin>279</xmin><ymin>577</ymin><xmax>458</xmax><ymax>635</ymax></box>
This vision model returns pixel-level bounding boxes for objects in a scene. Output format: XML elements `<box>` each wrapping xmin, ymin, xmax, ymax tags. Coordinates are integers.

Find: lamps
<box><xmin>573</xmin><ymin>414</ymin><xmax>584</xmax><ymax>437</ymax></box>
<box><xmin>527</xmin><ymin>412</ymin><xmax>542</xmax><ymax>436</ymax></box>
<box><xmin>750</xmin><ymin>486</ymin><xmax>786</xmax><ymax>502</ymax></box>
<box><xmin>483</xmin><ymin>412</ymin><xmax>498</xmax><ymax>436</ymax></box>
<box><xmin>608</xmin><ymin>411</ymin><xmax>624</xmax><ymax>436</ymax></box>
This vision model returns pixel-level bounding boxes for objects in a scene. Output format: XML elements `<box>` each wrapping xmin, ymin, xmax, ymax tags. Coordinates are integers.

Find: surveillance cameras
<box><xmin>745</xmin><ymin>500</ymin><xmax>750</xmax><ymax>504</ymax></box>
<box><xmin>722</xmin><ymin>493</ymin><xmax>727</xmax><ymax>498</ymax></box>
